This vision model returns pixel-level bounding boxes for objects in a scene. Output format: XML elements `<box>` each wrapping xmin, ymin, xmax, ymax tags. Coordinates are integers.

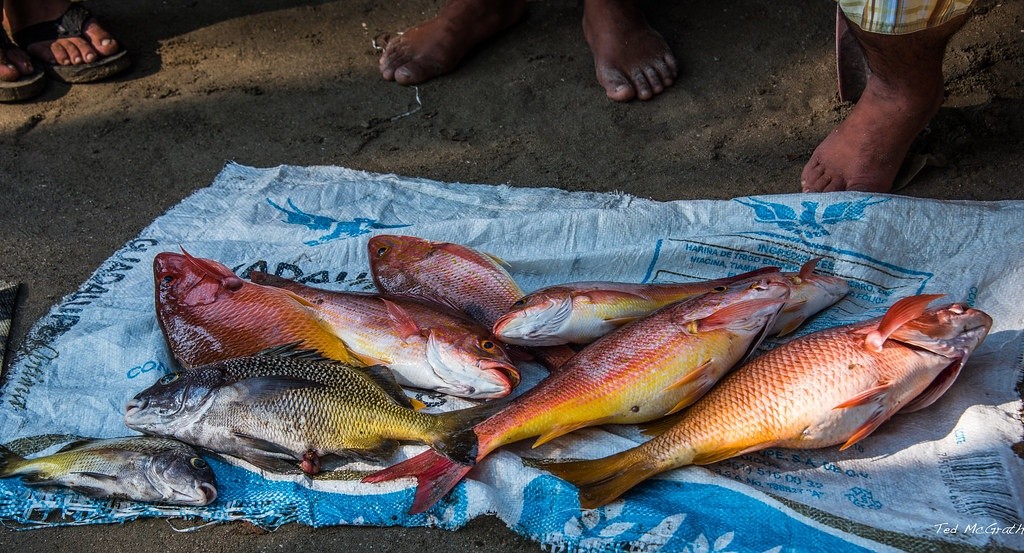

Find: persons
<box><xmin>0</xmin><ymin>0</ymin><xmax>132</xmax><ymax>103</ymax></box>
<box><xmin>379</xmin><ymin>0</ymin><xmax>681</xmax><ymax>102</ymax></box>
<box><xmin>797</xmin><ymin>0</ymin><xmax>1000</xmax><ymax>195</ymax></box>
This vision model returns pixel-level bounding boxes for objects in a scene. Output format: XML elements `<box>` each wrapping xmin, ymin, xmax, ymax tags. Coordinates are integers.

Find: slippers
<box><xmin>0</xmin><ymin>33</ymin><xmax>46</xmax><ymax>101</ymax></box>
<box><xmin>10</xmin><ymin>4</ymin><xmax>130</xmax><ymax>85</ymax></box>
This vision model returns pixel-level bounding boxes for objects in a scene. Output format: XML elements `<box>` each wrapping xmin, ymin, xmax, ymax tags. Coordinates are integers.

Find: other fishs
<box><xmin>0</xmin><ymin>234</ymin><xmax>994</xmax><ymax>513</ymax></box>
